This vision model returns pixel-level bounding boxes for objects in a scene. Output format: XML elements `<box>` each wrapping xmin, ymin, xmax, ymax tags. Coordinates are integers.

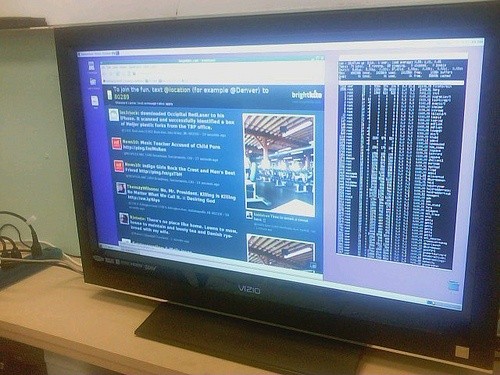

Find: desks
<box><xmin>0</xmin><ymin>249</ymin><xmax>500</xmax><ymax>375</ymax></box>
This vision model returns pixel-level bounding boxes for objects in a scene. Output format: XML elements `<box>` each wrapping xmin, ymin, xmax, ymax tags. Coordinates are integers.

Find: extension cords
<box><xmin>0</xmin><ymin>247</ymin><xmax>63</xmax><ymax>290</ymax></box>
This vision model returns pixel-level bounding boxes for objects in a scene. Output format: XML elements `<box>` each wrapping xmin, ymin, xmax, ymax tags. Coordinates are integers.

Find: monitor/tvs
<box><xmin>53</xmin><ymin>0</ymin><xmax>500</xmax><ymax>375</ymax></box>
